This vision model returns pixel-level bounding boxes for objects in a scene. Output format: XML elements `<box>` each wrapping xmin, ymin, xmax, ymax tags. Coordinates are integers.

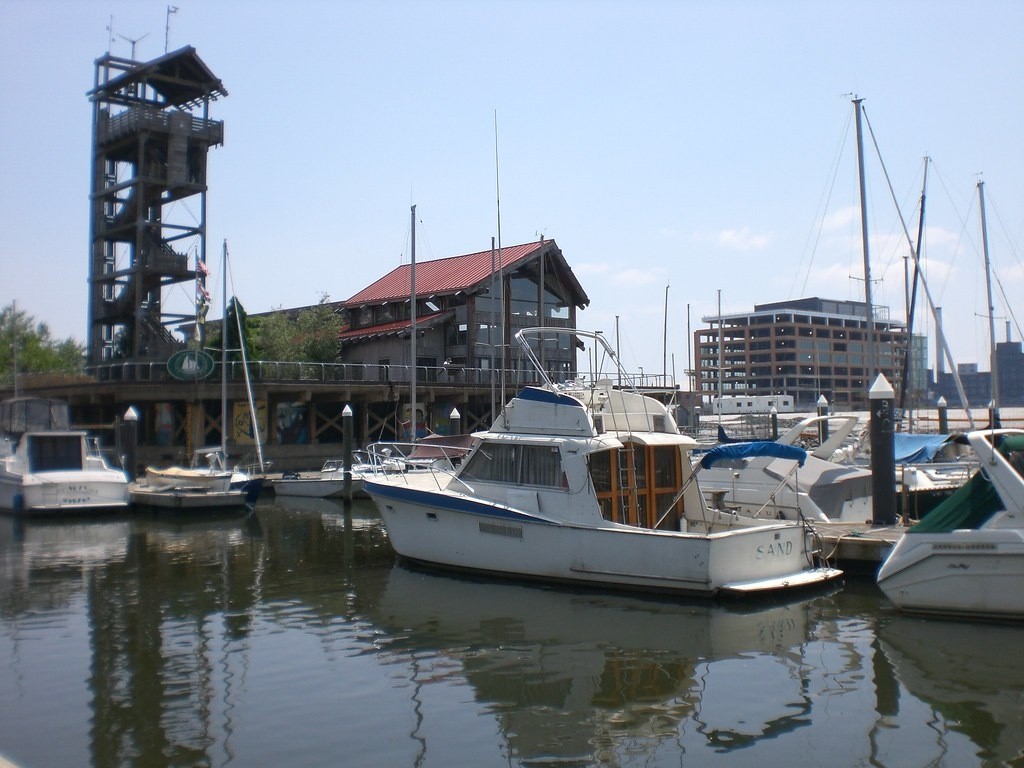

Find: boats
<box><xmin>362</xmin><ymin>327</ymin><xmax>844</xmax><ymax>602</ymax></box>
<box><xmin>0</xmin><ymin>431</ymin><xmax>129</xmax><ymax>516</ymax></box>
<box><xmin>695</xmin><ymin>413</ymin><xmax>896</xmax><ymax>564</ymax></box>
<box><xmin>317</xmin><ymin>447</ymin><xmax>435</xmax><ymax>483</ymax></box>
<box><xmin>271</xmin><ymin>471</ymin><xmax>363</xmax><ymax>497</ymax></box>
<box><xmin>132</xmin><ymin>465</ymin><xmax>260</xmax><ymax>511</ymax></box>
<box><xmin>877</xmin><ymin>429</ymin><xmax>1024</xmax><ymax>617</ymax></box>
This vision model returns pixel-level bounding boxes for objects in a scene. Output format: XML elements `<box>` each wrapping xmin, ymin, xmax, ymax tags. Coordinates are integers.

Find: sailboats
<box><xmin>201</xmin><ymin>242</ymin><xmax>264</xmax><ymax>506</ymax></box>
